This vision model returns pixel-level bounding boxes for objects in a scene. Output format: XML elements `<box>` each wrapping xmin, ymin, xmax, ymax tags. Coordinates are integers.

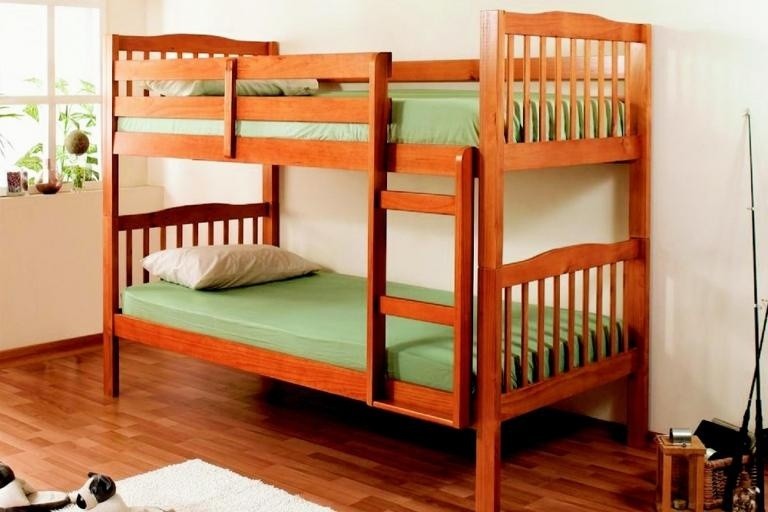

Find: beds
<box><xmin>99</xmin><ymin>11</ymin><xmax>662</xmax><ymax>512</ymax></box>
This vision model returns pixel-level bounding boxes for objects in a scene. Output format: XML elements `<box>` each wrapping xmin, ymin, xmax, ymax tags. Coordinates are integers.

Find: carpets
<box><xmin>39</xmin><ymin>456</ymin><xmax>333</xmax><ymax>510</ymax></box>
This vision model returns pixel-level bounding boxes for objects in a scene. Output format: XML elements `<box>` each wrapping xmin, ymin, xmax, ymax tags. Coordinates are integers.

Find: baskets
<box><xmin>705</xmin><ymin>418</ymin><xmax>759</xmax><ymax>510</ymax></box>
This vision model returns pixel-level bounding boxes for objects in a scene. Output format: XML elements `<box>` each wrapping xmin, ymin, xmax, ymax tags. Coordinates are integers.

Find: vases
<box><xmin>7</xmin><ymin>164</ymin><xmax>23</xmax><ymax>194</ymax></box>
<box><xmin>67</xmin><ymin>155</ymin><xmax>86</xmax><ymax>192</ymax></box>
<box><xmin>34</xmin><ymin>155</ymin><xmax>63</xmax><ymax>192</ymax></box>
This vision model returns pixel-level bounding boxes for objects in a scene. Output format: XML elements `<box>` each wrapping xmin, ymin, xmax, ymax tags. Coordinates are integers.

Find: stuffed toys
<box><xmin>75</xmin><ymin>470</ymin><xmax>174</xmax><ymax>512</ymax></box>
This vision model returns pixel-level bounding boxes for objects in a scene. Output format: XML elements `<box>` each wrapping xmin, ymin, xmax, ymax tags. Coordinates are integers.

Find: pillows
<box><xmin>144</xmin><ymin>55</ymin><xmax>317</xmax><ymax>98</ymax></box>
<box><xmin>138</xmin><ymin>243</ymin><xmax>320</xmax><ymax>293</ymax></box>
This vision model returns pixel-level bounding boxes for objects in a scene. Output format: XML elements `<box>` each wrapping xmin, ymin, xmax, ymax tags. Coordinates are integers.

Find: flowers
<box><xmin>63</xmin><ymin>129</ymin><xmax>90</xmax><ymax>192</ymax></box>
